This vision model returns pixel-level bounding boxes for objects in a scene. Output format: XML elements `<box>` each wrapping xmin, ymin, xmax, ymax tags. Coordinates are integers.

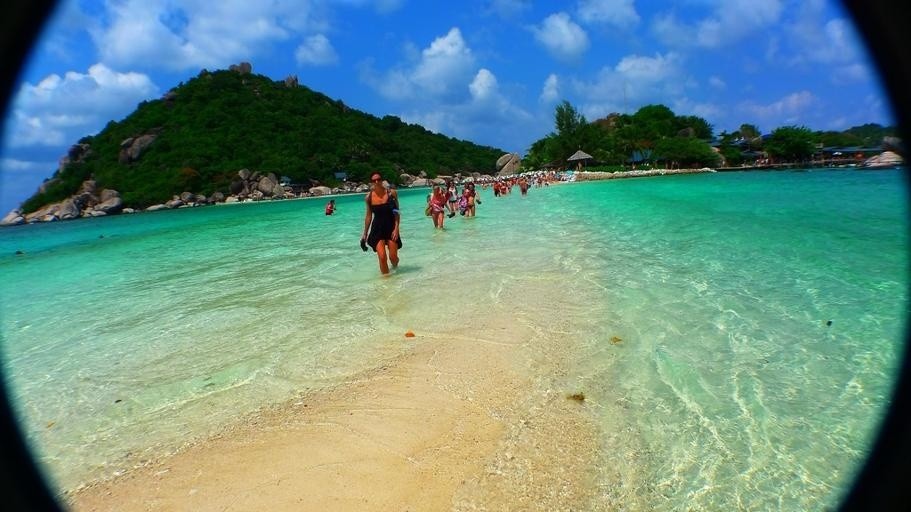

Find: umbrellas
<box><xmin>567</xmin><ymin>149</ymin><xmax>593</xmax><ymax>170</ymax></box>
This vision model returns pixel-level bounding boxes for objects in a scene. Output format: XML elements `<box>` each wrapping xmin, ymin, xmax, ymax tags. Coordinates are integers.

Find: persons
<box><xmin>424</xmin><ymin>167</ymin><xmax>561</xmax><ymax>232</ymax></box>
<box><xmin>359</xmin><ymin>171</ymin><xmax>403</xmax><ymax>275</ymax></box>
<box><xmin>325</xmin><ymin>199</ymin><xmax>337</xmax><ymax>215</ymax></box>
